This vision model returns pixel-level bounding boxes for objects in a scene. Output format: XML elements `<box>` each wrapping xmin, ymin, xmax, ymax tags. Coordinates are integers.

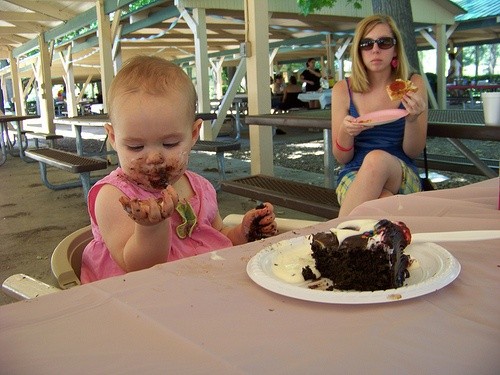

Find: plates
<box><xmin>352</xmin><ymin>109</ymin><xmax>409</xmax><ymax>127</ymax></box>
<box><xmin>246</xmin><ymin>231</ymin><xmax>461</xmax><ymax>304</ymax></box>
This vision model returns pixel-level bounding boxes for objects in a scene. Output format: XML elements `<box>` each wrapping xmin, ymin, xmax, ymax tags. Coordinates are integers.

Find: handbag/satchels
<box><xmin>421</xmin><ymin>178</ymin><xmax>437</xmax><ymax>191</ymax></box>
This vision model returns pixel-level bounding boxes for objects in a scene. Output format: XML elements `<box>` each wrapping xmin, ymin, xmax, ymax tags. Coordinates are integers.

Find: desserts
<box><xmin>245</xmin><ymin>204</ymin><xmax>268</xmax><ymax>241</ymax></box>
<box><xmin>302</xmin><ymin>218</ymin><xmax>412</xmax><ymax>292</ymax></box>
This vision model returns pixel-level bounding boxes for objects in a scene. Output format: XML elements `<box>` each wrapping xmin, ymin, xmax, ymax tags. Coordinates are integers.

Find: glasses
<box><xmin>360</xmin><ymin>37</ymin><xmax>396</xmax><ymax>50</ymax></box>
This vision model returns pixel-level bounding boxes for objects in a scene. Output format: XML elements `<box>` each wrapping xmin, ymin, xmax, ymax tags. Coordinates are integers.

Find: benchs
<box><xmin>24</xmin><ymin>147</ymin><xmax>108</xmax><ymax>203</ymax></box>
<box><xmin>192</xmin><ymin>140</ymin><xmax>240</xmax><ymax>178</ymax></box>
<box><xmin>21</xmin><ymin>130</ymin><xmax>63</xmax><ymax>163</ymax></box>
<box><xmin>416</xmin><ymin>152</ymin><xmax>500</xmax><ymax>176</ymax></box>
<box><xmin>447</xmin><ymin>97</ymin><xmax>468</xmax><ymax>109</ymax></box>
<box><xmin>218</xmin><ymin>175</ymin><xmax>341</xmax><ymax>219</ymax></box>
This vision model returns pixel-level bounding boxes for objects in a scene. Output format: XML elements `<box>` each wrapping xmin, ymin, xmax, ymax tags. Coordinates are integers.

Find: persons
<box><xmin>81</xmin><ymin>56</ymin><xmax>277</xmax><ymax>284</ymax></box>
<box><xmin>331</xmin><ymin>14</ymin><xmax>428</xmax><ymax>218</ymax></box>
<box><xmin>272</xmin><ymin>58</ymin><xmax>322</xmax><ymax>110</ymax></box>
<box><xmin>447</xmin><ymin>53</ymin><xmax>461</xmax><ymax>104</ymax></box>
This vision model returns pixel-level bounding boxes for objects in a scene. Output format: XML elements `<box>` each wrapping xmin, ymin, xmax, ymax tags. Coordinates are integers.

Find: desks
<box><xmin>298</xmin><ymin>89</ymin><xmax>332</xmax><ymax>109</ymax></box>
<box><xmin>246</xmin><ymin>109</ymin><xmax>500</xmax><ymax>190</ymax></box>
<box><xmin>222</xmin><ymin>94</ymin><xmax>248</xmax><ymax>140</ymax></box>
<box><xmin>0</xmin><ymin>115</ymin><xmax>40</xmax><ymax>163</ymax></box>
<box><xmin>447</xmin><ymin>83</ymin><xmax>500</xmax><ymax>109</ymax></box>
<box><xmin>0</xmin><ymin>177</ymin><xmax>500</xmax><ymax>375</ymax></box>
<box><xmin>51</xmin><ymin>113</ymin><xmax>218</xmax><ymax>203</ymax></box>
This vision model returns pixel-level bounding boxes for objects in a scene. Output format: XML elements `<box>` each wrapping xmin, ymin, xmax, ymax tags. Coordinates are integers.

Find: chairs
<box><xmin>1</xmin><ymin>214</ymin><xmax>324</xmax><ymax>301</ymax></box>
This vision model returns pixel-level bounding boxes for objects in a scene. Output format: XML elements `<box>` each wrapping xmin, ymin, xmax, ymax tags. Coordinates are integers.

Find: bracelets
<box><xmin>336</xmin><ymin>139</ymin><xmax>355</xmax><ymax>153</ymax></box>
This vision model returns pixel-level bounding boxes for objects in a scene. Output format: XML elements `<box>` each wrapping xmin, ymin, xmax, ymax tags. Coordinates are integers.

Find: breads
<box><xmin>387</xmin><ymin>78</ymin><xmax>418</xmax><ymax>101</ymax></box>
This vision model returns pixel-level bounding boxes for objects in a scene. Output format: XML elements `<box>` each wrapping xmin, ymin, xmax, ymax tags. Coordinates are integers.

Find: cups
<box><xmin>481</xmin><ymin>93</ymin><xmax>500</xmax><ymax>126</ymax></box>
